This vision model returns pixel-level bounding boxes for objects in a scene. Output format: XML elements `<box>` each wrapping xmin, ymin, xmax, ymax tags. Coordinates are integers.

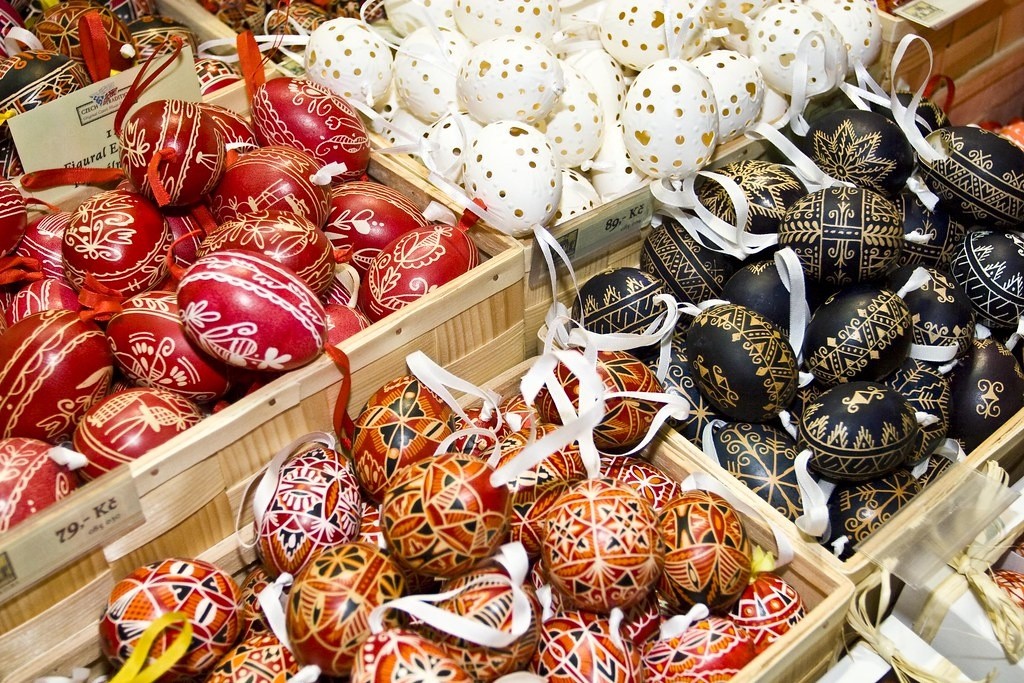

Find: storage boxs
<box><xmin>0</xmin><ymin>0</ymin><xmax>1024</xmax><ymax>682</ymax></box>
